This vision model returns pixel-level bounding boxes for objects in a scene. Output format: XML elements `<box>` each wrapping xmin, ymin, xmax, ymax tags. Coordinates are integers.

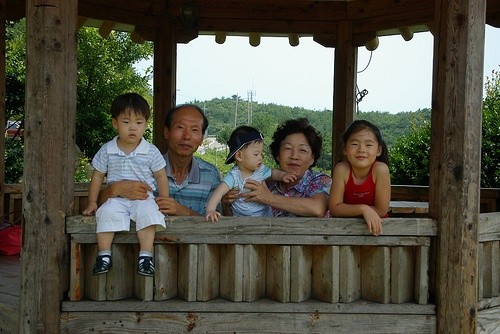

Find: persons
<box><xmin>83</xmin><ymin>94</ymin><xmax>168</xmax><ymax>277</ymax></box>
<box><xmin>206</xmin><ymin>128</ymin><xmax>298</xmax><ymax>223</ymax></box>
<box><xmin>223</xmin><ymin>118</ymin><xmax>334</xmax><ymax>218</ymax></box>
<box><xmin>330</xmin><ymin>119</ymin><xmax>391</xmax><ymax>236</ymax></box>
<box><xmin>153</xmin><ymin>105</ymin><xmax>223</xmax><ymax>216</ymax></box>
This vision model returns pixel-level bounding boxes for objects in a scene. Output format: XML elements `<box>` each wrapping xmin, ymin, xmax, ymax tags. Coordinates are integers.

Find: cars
<box><xmin>5</xmin><ymin>120</ymin><xmax>24</xmax><ymax>139</ymax></box>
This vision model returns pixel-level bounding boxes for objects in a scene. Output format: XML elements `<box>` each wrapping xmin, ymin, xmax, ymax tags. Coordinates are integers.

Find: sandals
<box><xmin>136</xmin><ymin>257</ymin><xmax>155</xmax><ymax>277</ymax></box>
<box><xmin>92</xmin><ymin>254</ymin><xmax>111</xmax><ymax>275</ymax></box>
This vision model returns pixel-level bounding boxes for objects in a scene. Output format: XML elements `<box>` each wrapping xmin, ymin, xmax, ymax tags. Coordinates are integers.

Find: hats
<box><xmin>224</xmin><ymin>126</ymin><xmax>264</xmax><ymax>165</ymax></box>
